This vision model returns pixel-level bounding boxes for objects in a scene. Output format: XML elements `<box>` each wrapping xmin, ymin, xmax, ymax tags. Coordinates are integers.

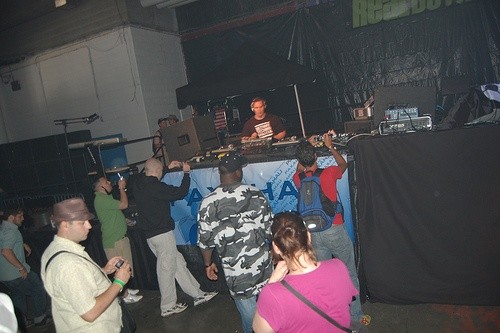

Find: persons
<box><xmin>89</xmin><ymin>171</ymin><xmax>143</xmax><ymax>303</ymax></box>
<box><xmin>197</xmin><ymin>152</ymin><xmax>282</xmax><ymax>333</ymax></box>
<box><xmin>241</xmin><ymin>97</ymin><xmax>286</xmax><ymax>143</ymax></box>
<box><xmin>0</xmin><ymin>202</ymin><xmax>57</xmax><ymax>331</ymax></box>
<box><xmin>151</xmin><ymin>114</ymin><xmax>180</xmax><ymax>167</ymax></box>
<box><xmin>133</xmin><ymin>158</ymin><xmax>219</xmax><ymax>317</ymax></box>
<box><xmin>251</xmin><ymin>210</ymin><xmax>358</xmax><ymax>333</ymax></box>
<box><xmin>39</xmin><ymin>197</ymin><xmax>137</xmax><ymax>332</ymax></box>
<box><xmin>293</xmin><ymin>134</ymin><xmax>371</xmax><ymax>333</ymax></box>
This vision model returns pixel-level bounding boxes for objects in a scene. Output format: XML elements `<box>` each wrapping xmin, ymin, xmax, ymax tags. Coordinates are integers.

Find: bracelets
<box><xmin>328</xmin><ymin>145</ymin><xmax>334</xmax><ymax>151</ymax></box>
<box><xmin>117</xmin><ymin>186</ymin><xmax>128</xmax><ymax>192</ymax></box>
<box><xmin>112</xmin><ymin>279</ymin><xmax>127</xmax><ymax>294</ymax></box>
<box><xmin>204</xmin><ymin>261</ymin><xmax>214</xmax><ymax>269</ymax></box>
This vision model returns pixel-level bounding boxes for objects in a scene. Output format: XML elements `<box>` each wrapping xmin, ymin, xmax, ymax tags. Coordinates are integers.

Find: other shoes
<box><xmin>34</xmin><ymin>317</ymin><xmax>54</xmax><ymax>329</ymax></box>
<box><xmin>352</xmin><ymin>315</ymin><xmax>371</xmax><ymax>333</ymax></box>
<box><xmin>123</xmin><ymin>294</ymin><xmax>143</xmax><ymax>304</ymax></box>
<box><xmin>128</xmin><ymin>288</ymin><xmax>139</xmax><ymax>295</ymax></box>
<box><xmin>24</xmin><ymin>320</ymin><xmax>34</xmax><ymax>329</ymax></box>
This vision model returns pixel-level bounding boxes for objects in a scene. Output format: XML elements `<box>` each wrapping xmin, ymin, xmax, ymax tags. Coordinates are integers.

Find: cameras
<box><xmin>316</xmin><ymin>135</ymin><xmax>324</xmax><ymax>141</ymax></box>
<box><xmin>115</xmin><ymin>260</ymin><xmax>131</xmax><ymax>271</ymax></box>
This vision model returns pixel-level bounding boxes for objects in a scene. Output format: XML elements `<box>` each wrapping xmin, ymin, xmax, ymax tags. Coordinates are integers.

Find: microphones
<box><xmin>86</xmin><ymin>114</ymin><xmax>96</xmax><ymax>120</ymax></box>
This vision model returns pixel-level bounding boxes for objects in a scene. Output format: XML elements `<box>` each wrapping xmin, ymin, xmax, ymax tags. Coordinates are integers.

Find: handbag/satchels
<box><xmin>119</xmin><ymin>303</ymin><xmax>136</xmax><ymax>333</ymax></box>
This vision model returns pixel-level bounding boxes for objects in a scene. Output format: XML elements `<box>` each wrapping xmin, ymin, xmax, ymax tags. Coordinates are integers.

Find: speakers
<box><xmin>344</xmin><ymin>119</ymin><xmax>374</xmax><ymax>136</ymax></box>
<box><xmin>163</xmin><ymin>116</ymin><xmax>219</xmax><ymax>163</ymax></box>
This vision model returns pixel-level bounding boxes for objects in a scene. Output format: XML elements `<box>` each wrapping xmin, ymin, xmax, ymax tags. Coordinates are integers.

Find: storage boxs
<box><xmin>161</xmin><ymin>114</ymin><xmax>221</xmax><ymax>168</ymax></box>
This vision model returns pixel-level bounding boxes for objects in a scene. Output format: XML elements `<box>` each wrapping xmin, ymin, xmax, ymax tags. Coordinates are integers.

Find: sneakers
<box><xmin>161</xmin><ymin>303</ymin><xmax>188</xmax><ymax>317</ymax></box>
<box><xmin>193</xmin><ymin>291</ymin><xmax>218</xmax><ymax>306</ymax></box>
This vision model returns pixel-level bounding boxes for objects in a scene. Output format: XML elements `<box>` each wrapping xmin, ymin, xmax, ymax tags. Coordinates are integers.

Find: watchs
<box><xmin>183</xmin><ymin>170</ymin><xmax>192</xmax><ymax>175</ymax></box>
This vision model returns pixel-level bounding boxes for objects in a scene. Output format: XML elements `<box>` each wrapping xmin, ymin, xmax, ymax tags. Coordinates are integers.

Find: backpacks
<box><xmin>298</xmin><ymin>169</ymin><xmax>344</xmax><ymax>232</ymax></box>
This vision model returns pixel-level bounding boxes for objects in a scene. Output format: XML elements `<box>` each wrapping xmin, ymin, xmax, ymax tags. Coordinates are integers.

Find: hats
<box><xmin>219</xmin><ymin>153</ymin><xmax>248</xmax><ymax>173</ymax></box>
<box><xmin>158</xmin><ymin>118</ymin><xmax>167</xmax><ymax>124</ymax></box>
<box><xmin>168</xmin><ymin>115</ymin><xmax>178</xmax><ymax>121</ymax></box>
<box><xmin>53</xmin><ymin>198</ymin><xmax>95</xmax><ymax>225</ymax></box>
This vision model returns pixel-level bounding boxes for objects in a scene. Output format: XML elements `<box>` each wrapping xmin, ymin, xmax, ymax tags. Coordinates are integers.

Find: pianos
<box><xmin>67</xmin><ymin>137</ymin><xmax>130</xmax><ymax>179</ymax></box>
<box><xmin>210</xmin><ymin>138</ymin><xmax>300</xmax><ymax>156</ymax></box>
